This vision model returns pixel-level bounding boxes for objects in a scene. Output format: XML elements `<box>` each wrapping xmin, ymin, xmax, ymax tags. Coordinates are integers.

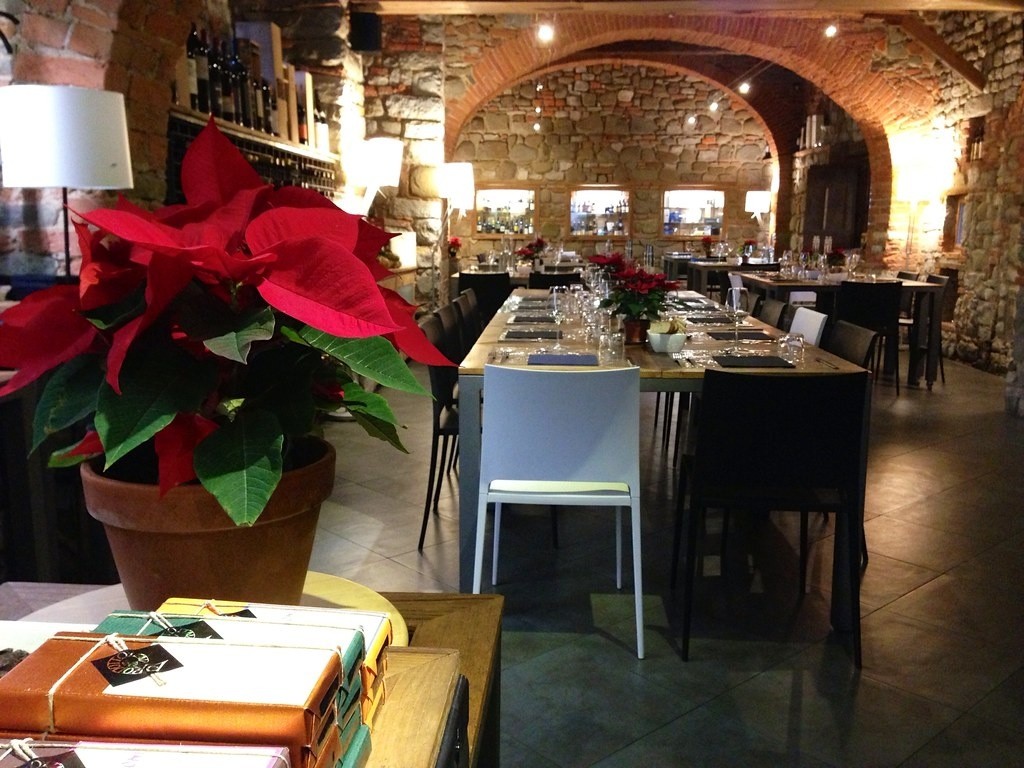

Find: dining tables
<box><xmin>451</xmin><ymin>258</ymin><xmax>588</xmax><ymax>289</ymax></box>
<box><xmin>688</xmin><ymin>260</ymin><xmax>781</xmax><ymax>297</ymax></box>
<box><xmin>653</xmin><ymin>271</ymin><xmax>946</xmax><ymax>471</ymax></box>
<box><xmin>663</xmin><ymin>251</ymin><xmax>708</xmax><ymax>280</ymax></box>
<box><xmin>457</xmin><ymin>288</ymin><xmax>874</xmax><ymax>630</ymax></box>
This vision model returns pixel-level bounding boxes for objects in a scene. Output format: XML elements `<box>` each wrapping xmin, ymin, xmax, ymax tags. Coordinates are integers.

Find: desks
<box><xmin>0</xmin><ymin>577</ymin><xmax>507</xmax><ymax>768</ymax></box>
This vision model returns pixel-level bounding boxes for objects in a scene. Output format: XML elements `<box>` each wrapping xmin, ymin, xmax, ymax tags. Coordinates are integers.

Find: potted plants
<box><xmin>587</xmin><ymin>252</ymin><xmax>680</xmax><ymax>344</ymax></box>
<box><xmin>0</xmin><ymin>112</ymin><xmax>463</xmax><ymax>602</ymax></box>
<box><xmin>448</xmin><ymin>237</ymin><xmax>462</xmax><ymax>258</ymax></box>
<box><xmin>825</xmin><ymin>249</ymin><xmax>848</xmax><ymax>282</ymax></box>
<box><xmin>516</xmin><ymin>247</ymin><xmax>536</xmax><ymax>263</ymax></box>
<box><xmin>701</xmin><ymin>236</ymin><xmax>711</xmax><ymax>257</ymax></box>
<box><xmin>528</xmin><ymin>237</ymin><xmax>547</xmax><ymax>265</ymax></box>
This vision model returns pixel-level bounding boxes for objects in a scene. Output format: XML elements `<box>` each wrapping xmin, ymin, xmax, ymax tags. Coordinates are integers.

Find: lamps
<box><xmin>0</xmin><ymin>83</ymin><xmax>136</xmax><ymax>281</ymax></box>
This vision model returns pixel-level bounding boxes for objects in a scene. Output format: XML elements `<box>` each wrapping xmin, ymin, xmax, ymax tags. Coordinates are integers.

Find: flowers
<box><xmin>745</xmin><ymin>239</ymin><xmax>758</xmax><ymax>246</ymax></box>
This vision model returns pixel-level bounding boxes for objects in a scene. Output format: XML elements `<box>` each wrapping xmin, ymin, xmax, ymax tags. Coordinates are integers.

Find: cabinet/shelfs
<box><xmin>170</xmin><ymin>20</ymin><xmax>341</xmax><ymax>204</ymax></box>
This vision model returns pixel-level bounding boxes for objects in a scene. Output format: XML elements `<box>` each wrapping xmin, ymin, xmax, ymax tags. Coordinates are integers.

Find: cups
<box><xmin>798</xmin><ymin>114</ymin><xmax>826</xmax><ymax>151</ymax></box>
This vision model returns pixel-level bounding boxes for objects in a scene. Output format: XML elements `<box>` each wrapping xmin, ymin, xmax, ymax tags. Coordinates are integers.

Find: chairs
<box><xmin>871</xmin><ymin>269</ymin><xmax>920</xmax><ymax>382</ymax></box>
<box><xmin>670</xmin><ymin>369</ymin><xmax>870</xmax><ymax>675</ymax></box>
<box><xmin>939</xmin><ymin>266</ymin><xmax>960</xmax><ymax>322</ymax></box>
<box><xmin>472</xmin><ymin>363</ymin><xmax>646</xmax><ymax>662</ymax></box>
<box><xmin>415</xmin><ymin>289</ymin><xmax>561</xmax><ymax>555</ymax></box>
<box><xmin>895</xmin><ymin>273</ymin><xmax>950</xmax><ymax>396</ymax></box>
<box><xmin>529</xmin><ymin>272</ymin><xmax>581</xmax><ymax>289</ymax></box>
<box><xmin>458</xmin><ymin>272</ymin><xmax>512</xmax><ymax>324</ymax></box>
<box><xmin>726</xmin><ymin>287</ymin><xmax>880</xmax><ymax>369</ymax></box>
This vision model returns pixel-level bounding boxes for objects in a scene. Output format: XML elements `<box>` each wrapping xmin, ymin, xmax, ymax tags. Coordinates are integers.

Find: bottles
<box><xmin>970</xmin><ymin>125</ymin><xmax>986</xmax><ymax>162</ymax></box>
<box><xmin>165</xmin><ymin>22</ymin><xmax>337</xmax><ymax>200</ymax></box>
<box><xmin>475</xmin><ymin>196</ymin><xmax>630</xmax><ymax>238</ymax></box>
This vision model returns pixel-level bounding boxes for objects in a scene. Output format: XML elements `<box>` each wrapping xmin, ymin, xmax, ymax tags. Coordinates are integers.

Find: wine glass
<box><xmin>476</xmin><ymin>242</ymin><xmax>869</xmax><ymax>369</ymax></box>
